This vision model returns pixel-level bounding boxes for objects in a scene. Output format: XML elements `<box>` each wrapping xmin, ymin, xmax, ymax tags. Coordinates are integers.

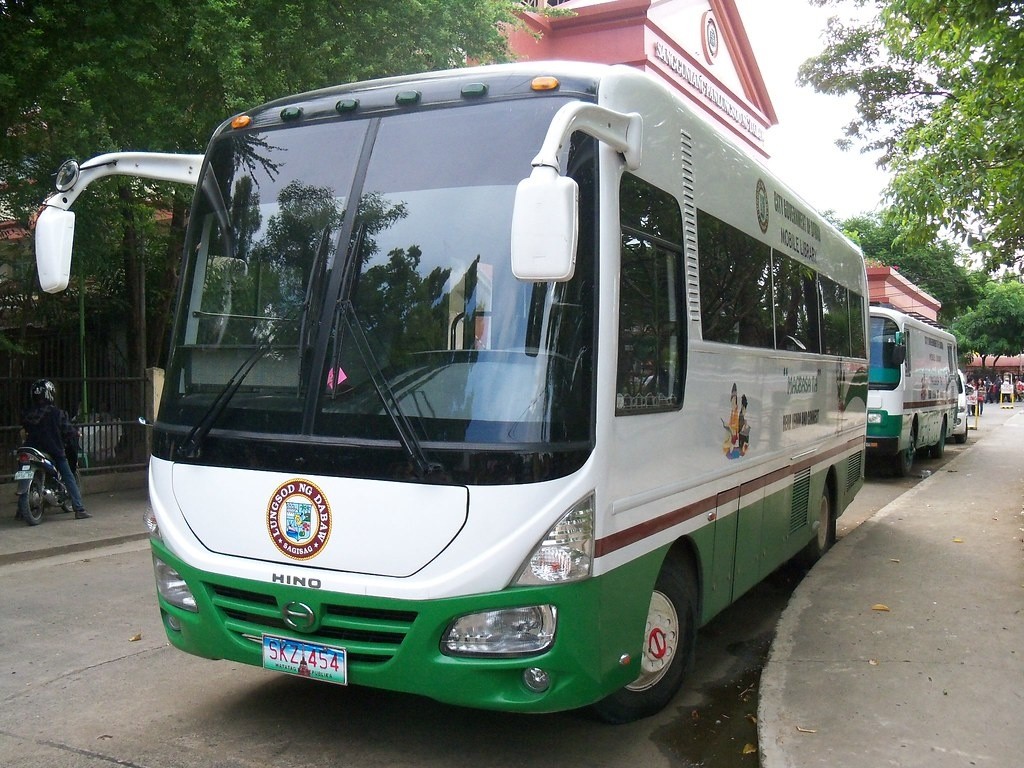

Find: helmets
<box><xmin>30</xmin><ymin>379</ymin><xmax>56</xmax><ymax>405</ymax></box>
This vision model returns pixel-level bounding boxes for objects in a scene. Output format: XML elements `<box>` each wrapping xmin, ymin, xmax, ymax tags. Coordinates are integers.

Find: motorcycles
<box><xmin>14</xmin><ymin>417</ymin><xmax>82</xmax><ymax>525</ymax></box>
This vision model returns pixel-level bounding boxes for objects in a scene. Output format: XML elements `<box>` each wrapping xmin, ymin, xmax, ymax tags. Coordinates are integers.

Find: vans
<box><xmin>952</xmin><ymin>368</ymin><xmax>973</xmax><ymax>443</ymax></box>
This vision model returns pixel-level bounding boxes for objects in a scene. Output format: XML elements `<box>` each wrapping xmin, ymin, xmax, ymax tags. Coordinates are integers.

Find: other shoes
<box><xmin>972</xmin><ymin>414</ymin><xmax>974</xmax><ymax>416</ymax></box>
<box><xmin>985</xmin><ymin>398</ymin><xmax>1023</xmax><ymax>403</ymax></box>
<box><xmin>969</xmin><ymin>414</ymin><xmax>970</xmax><ymax>415</ymax></box>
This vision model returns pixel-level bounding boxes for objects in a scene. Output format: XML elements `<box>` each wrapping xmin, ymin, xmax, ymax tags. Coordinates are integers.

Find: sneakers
<box><xmin>14</xmin><ymin>510</ymin><xmax>23</xmax><ymax>521</ymax></box>
<box><xmin>76</xmin><ymin>510</ymin><xmax>92</xmax><ymax>518</ymax></box>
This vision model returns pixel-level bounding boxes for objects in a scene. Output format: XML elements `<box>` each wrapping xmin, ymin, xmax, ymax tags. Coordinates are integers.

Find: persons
<box><xmin>714</xmin><ymin>312</ymin><xmax>783</xmax><ymax>350</ymax></box>
<box><xmin>966</xmin><ymin>374</ymin><xmax>1024</xmax><ymax>416</ymax></box>
<box><xmin>15</xmin><ymin>378</ymin><xmax>93</xmax><ymax>521</ymax></box>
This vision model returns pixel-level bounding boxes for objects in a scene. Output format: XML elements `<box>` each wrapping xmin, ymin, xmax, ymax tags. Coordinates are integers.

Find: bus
<box><xmin>31</xmin><ymin>58</ymin><xmax>868</xmax><ymax>723</ymax></box>
<box><xmin>866</xmin><ymin>302</ymin><xmax>961</xmax><ymax>477</ymax></box>
